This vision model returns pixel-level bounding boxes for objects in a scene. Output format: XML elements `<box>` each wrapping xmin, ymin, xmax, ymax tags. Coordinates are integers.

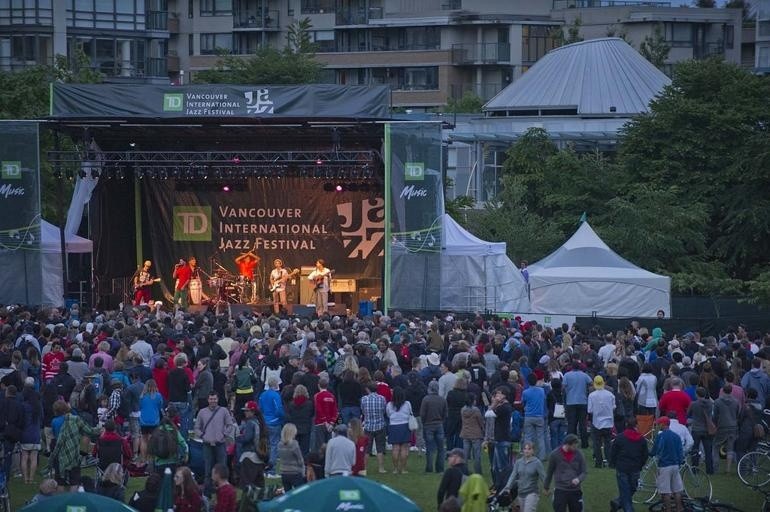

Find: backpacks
<box><xmin>507</xmin><ymin>404</ymin><xmax>523</xmax><ymax>442</ymax></box>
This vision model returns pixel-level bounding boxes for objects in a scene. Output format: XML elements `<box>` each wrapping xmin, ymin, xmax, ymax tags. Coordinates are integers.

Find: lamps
<box><xmin>324</xmin><ymin>182</ymin><xmax>367</xmax><ymax>193</ymax></box>
<box><xmin>176</xmin><ymin>182</ymin><xmax>243</xmax><ymax>192</ymax></box>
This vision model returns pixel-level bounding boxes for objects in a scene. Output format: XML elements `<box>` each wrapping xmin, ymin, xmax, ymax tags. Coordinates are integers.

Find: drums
<box><xmin>219</xmin><ymin>280</ymin><xmax>243</xmax><ymax>304</ymax></box>
<box><xmin>189</xmin><ymin>278</ymin><xmax>203</xmax><ymax>304</ymax></box>
<box><xmin>174</xmin><ymin>278</ymin><xmax>182</xmax><ymax>304</ymax></box>
<box><xmin>206</xmin><ymin>275</ymin><xmax>224</xmax><ymax>288</ymax></box>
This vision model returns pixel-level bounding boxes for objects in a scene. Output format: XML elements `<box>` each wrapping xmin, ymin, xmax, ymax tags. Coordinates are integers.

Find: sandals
<box><xmin>401</xmin><ymin>469</ymin><xmax>409</xmax><ymax>474</ymax></box>
<box><xmin>379</xmin><ymin>469</ymin><xmax>388</xmax><ymax>474</ymax></box>
<box><xmin>393</xmin><ymin>469</ymin><xmax>399</xmax><ymax>474</ymax></box>
<box><xmin>25</xmin><ymin>480</ymin><xmax>35</xmax><ymax>485</ymax></box>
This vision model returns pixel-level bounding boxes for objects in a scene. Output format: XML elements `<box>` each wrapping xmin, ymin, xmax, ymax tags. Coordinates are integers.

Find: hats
<box><xmin>374</xmin><ymin>311</ymin><xmax>382</xmax><ymax>316</ymax></box>
<box><xmin>694</xmin><ymin>352</ymin><xmax>703</xmax><ymax>362</ymax></box>
<box><xmin>268</xmin><ymin>374</ymin><xmax>282</xmax><ymax>385</ymax></box>
<box><xmin>275</xmin><ymin>259</ymin><xmax>282</xmax><ymax>266</ymax></box>
<box><xmin>446</xmin><ymin>449</ymin><xmax>465</xmax><ymax>457</ymax></box>
<box><xmin>497</xmin><ymin>360</ymin><xmax>510</xmax><ymax>369</ymax></box>
<box><xmin>682</xmin><ymin>332</ymin><xmax>695</xmax><ymax>339</ymax></box>
<box><xmin>333</xmin><ymin>424</ymin><xmax>347</xmax><ymax>432</ymax></box>
<box><xmin>540</xmin><ymin>355</ymin><xmax>550</xmax><ymax>364</ymax></box>
<box><xmin>682</xmin><ymin>357</ymin><xmax>692</xmax><ymax>365</ymax></box>
<box><xmin>428</xmin><ymin>381</ymin><xmax>439</xmax><ymax>395</ymax></box>
<box><xmin>73</xmin><ymin>320</ymin><xmax>81</xmax><ymax>327</ymax></box>
<box><xmin>250</xmin><ymin>325</ymin><xmax>261</xmax><ymax>335</ymax></box>
<box><xmin>428</xmin><ymin>353</ymin><xmax>441</xmax><ymax>366</ymax></box>
<box><xmin>241</xmin><ymin>401</ymin><xmax>257</xmax><ymax>411</ymax></box>
<box><xmin>144</xmin><ymin>261</ymin><xmax>152</xmax><ymax>266</ymax></box>
<box><xmin>380</xmin><ymin>316</ymin><xmax>390</xmax><ymax>324</ymax></box>
<box><xmin>593</xmin><ymin>375</ymin><xmax>604</xmax><ymax>389</ymax></box>
<box><xmin>669</xmin><ymin>340</ymin><xmax>679</xmax><ymax>347</ymax></box>
<box><xmin>657</xmin><ymin>416</ymin><xmax>671</xmax><ymax>426</ymax></box>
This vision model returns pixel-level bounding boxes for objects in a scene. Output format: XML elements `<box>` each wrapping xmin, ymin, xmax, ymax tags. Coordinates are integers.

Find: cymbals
<box><xmin>214</xmin><ymin>268</ymin><xmax>229</xmax><ymax>279</ymax></box>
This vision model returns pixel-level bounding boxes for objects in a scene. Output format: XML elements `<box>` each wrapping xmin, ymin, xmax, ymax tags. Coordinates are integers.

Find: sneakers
<box><xmin>610</xmin><ymin>500</ymin><xmax>618</xmax><ymax>512</ymax></box>
<box><xmin>582</xmin><ymin>442</ymin><xmax>590</xmax><ymax>448</ymax></box>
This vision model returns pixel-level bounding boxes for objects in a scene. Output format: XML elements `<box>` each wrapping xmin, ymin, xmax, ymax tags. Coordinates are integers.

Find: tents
<box><xmin>39</xmin><ymin>217</ymin><xmax>92</xmax><ymax>307</ymax></box>
<box><xmin>391</xmin><ymin>214</ymin><xmax>529</xmax><ymax>313</ymax></box>
<box><xmin>518</xmin><ymin>212</ymin><xmax>671</xmax><ymax>317</ymax></box>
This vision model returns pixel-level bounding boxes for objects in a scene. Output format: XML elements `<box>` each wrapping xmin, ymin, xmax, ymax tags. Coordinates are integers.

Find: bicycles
<box><xmin>737</xmin><ymin>409</ymin><xmax>770</xmax><ymax>490</ymax></box>
<box><xmin>632</xmin><ymin>429</ymin><xmax>712</xmax><ymax>503</ymax></box>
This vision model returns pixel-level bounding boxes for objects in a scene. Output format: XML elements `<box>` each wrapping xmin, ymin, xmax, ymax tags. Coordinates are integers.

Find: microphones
<box><xmin>195</xmin><ymin>266</ymin><xmax>200</xmax><ymax>271</ymax></box>
<box><xmin>137</xmin><ymin>264</ymin><xmax>140</xmax><ymax>270</ymax></box>
<box><xmin>207</xmin><ymin>251</ymin><xmax>219</xmax><ymax>258</ymax></box>
<box><xmin>175</xmin><ymin>263</ymin><xmax>181</xmax><ymax>267</ymax></box>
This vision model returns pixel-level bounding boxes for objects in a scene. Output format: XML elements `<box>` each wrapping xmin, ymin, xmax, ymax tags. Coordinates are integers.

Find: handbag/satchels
<box><xmin>551</xmin><ymin>393</ymin><xmax>565</xmax><ymax>418</ymax></box>
<box><xmin>701</xmin><ymin>406</ymin><xmax>717</xmax><ymax>435</ymax></box>
<box><xmin>745</xmin><ymin>404</ymin><xmax>766</xmax><ymax>437</ymax></box>
<box><xmin>259</xmin><ymin>365</ymin><xmax>266</xmax><ymax>390</ymax></box>
<box><xmin>231</xmin><ymin>366</ymin><xmax>238</xmax><ymax>392</ymax></box>
<box><xmin>490</xmin><ymin>488</ymin><xmax>513</xmax><ymax>512</ymax></box>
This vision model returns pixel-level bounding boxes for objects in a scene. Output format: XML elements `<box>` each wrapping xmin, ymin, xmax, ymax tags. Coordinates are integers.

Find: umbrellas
<box><xmin>254</xmin><ymin>477</ymin><xmax>421</xmax><ymax>512</ymax></box>
<box><xmin>14</xmin><ymin>493</ymin><xmax>139</xmax><ymax>512</ymax></box>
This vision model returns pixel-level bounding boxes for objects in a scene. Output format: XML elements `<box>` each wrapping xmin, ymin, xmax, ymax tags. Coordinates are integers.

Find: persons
<box><xmin>131</xmin><ymin>356</ymin><xmax>151</xmax><ymax>382</ymax></box>
<box><xmin>481</xmin><ymin>393</ymin><xmax>499</xmax><ymax>462</ymax></box>
<box><xmin>92</xmin><ymin>420</ymin><xmax>133</xmax><ymax>470</ymax></box>
<box><xmin>324</xmin><ymin>426</ymin><xmax>355</xmax><ymax>477</ymax></box>
<box><xmin>208</xmin><ymin>359</ymin><xmax>228</xmax><ymax>410</ymax></box>
<box><xmin>405</xmin><ymin>374</ymin><xmax>422</xmax><ymax>451</ymax></box>
<box><xmin>167</xmin><ymin>353</ymin><xmax>191</xmax><ymax>437</ymax></box>
<box><xmin>522</xmin><ymin>374</ymin><xmax>546</xmax><ymax>461</ymax></box>
<box><xmin>90</xmin><ymin>311</ymin><xmax>130</xmax><ymax>387</ymax></box>
<box><xmin>587</xmin><ymin>374</ymin><xmax>617</xmax><ymax>467</ymax></box>
<box><xmin>740</xmin><ymin>359</ymin><xmax>770</xmax><ymax>409</ymax></box>
<box><xmin>194</xmin><ymin>390</ymin><xmax>234</xmax><ymax>490</ymax></box>
<box><xmin>130</xmin><ymin>295</ymin><xmax>212</xmax><ymax>356</ymax></box>
<box><xmin>445</xmin><ymin>378</ymin><xmax>470</xmax><ymax>449</ymax></box>
<box><xmin>546</xmin><ymin>377</ymin><xmax>565</xmax><ymax>448</ymax></box>
<box><xmin>125</xmin><ymin>373</ymin><xmax>145</xmax><ymax>461</ymax></box>
<box><xmin>606</xmin><ymin>416</ymin><xmax>649</xmax><ymax>511</ymax></box>
<box><xmin>447</xmin><ymin>314</ymin><xmax>507</xmax><ymax>385</ymax></box>
<box><xmin>210</xmin><ymin>462</ymin><xmax>237</xmax><ymax>512</ymax></box>
<box><xmin>507</xmin><ymin>370</ymin><xmax>524</xmax><ymax>441</ymax></box>
<box><xmin>42</xmin><ymin>401</ymin><xmax>102</xmax><ymax>492</ymax></box>
<box><xmin>347</xmin><ymin>420</ymin><xmax>369</xmax><ymax>476</ymax></box>
<box><xmin>648</xmin><ymin>416</ymin><xmax>685</xmax><ymax>512</ymax></box>
<box><xmin>277</xmin><ymin>423</ymin><xmax>307</xmax><ymax>491</ymax></box>
<box><xmin>421</xmin><ymin>381</ymin><xmax>449</xmax><ymax>473</ymax></box>
<box><xmin>634</xmin><ymin>363</ymin><xmax>659</xmax><ymax>415</ymax></box>
<box><xmin>42</xmin><ymin>384</ymin><xmax>60</xmax><ymax>457</ymax></box>
<box><xmin>257</xmin><ymin>377</ymin><xmax>286</xmax><ymax>467</ymax></box>
<box><xmin>361</xmin><ymin>311</ymin><xmax>445</xmax><ymax>381</ymax></box>
<box><xmin>0</xmin><ymin>384</ymin><xmax>23</xmax><ymax>483</ymax></box>
<box><xmin>458</xmin><ymin>393</ymin><xmax>486</xmax><ymax>474</ymax></box>
<box><xmin>658</xmin><ymin>411</ymin><xmax>694</xmax><ymax>454</ymax></box>
<box><xmin>96</xmin><ymin>463</ymin><xmax>128</xmax><ymax>504</ymax></box>
<box><xmin>96</xmin><ymin>395</ymin><xmax>113</xmax><ymax>428</ymax></box>
<box><xmin>735</xmin><ymin>387</ymin><xmax>766</xmax><ymax>472</ymax></box>
<box><xmin>437</xmin><ymin>448</ymin><xmax>469</xmax><ymax>511</ymax></box>
<box><xmin>287</xmin><ymin>386</ymin><xmax>314</xmax><ymax>436</ymax></box>
<box><xmin>439</xmin><ymin>497</ymin><xmax>462</xmax><ymax>511</ymax></box>
<box><xmin>128</xmin><ymin>260</ymin><xmax>155</xmax><ymax>306</ymax></box>
<box><xmin>613</xmin><ymin>378</ymin><xmax>637</xmax><ymax>432</ymax></box>
<box><xmin>384</xmin><ymin>386</ymin><xmax>419</xmax><ymax>475</ymax></box>
<box><xmin>193</xmin><ymin>357</ymin><xmax>213</xmax><ymax>411</ymax></box>
<box><xmin>272</xmin><ymin>313</ymin><xmax>360</xmax><ymax>380</ymax></box>
<box><xmin>502</xmin><ymin>316</ymin><xmax>591</xmax><ymax>375</ymax></box>
<box><xmin>687</xmin><ymin>387</ymin><xmax>715</xmax><ymax>475</ymax></box>
<box><xmin>269</xmin><ymin>259</ymin><xmax>289</xmax><ymax>314</ymax></box>
<box><xmin>234</xmin><ymin>249</ymin><xmax>261</xmax><ymax>304</ymax></box>
<box><xmin>173</xmin><ymin>258</ymin><xmax>191</xmax><ymax>312</ymax></box>
<box><xmin>591</xmin><ymin>321</ymin><xmax>658</xmax><ymax>375</ymax></box>
<box><xmin>139</xmin><ymin>377</ymin><xmax>165</xmax><ymax>456</ymax></box>
<box><xmin>359</xmin><ymin>383</ymin><xmax>389</xmax><ymax>473</ymax></box>
<box><xmin>28</xmin><ymin>480</ymin><xmax>58</xmax><ymax>504</ymax></box>
<box><xmin>234</xmin><ymin>401</ymin><xmax>269</xmax><ymax>483</ymax></box>
<box><xmin>233</xmin><ymin>354</ymin><xmax>256</xmax><ymax>425</ymax></box>
<box><xmin>306</xmin><ymin>431</ymin><xmax>330</xmax><ymax>479</ymax></box>
<box><xmin>719</xmin><ymin>372</ymin><xmax>745</xmax><ymax>411</ymax></box>
<box><xmin>187</xmin><ymin>257</ymin><xmax>200</xmax><ymax>304</ymax></box>
<box><xmin>151</xmin><ymin>359</ymin><xmax>168</xmax><ymax>399</ymax></box>
<box><xmin>103</xmin><ymin>380</ymin><xmax>123</xmax><ymax>420</ymax></box>
<box><xmin>129</xmin><ymin>474</ymin><xmax>161</xmax><ymax>512</ymax></box>
<box><xmin>16</xmin><ymin>382</ymin><xmax>43</xmax><ymax>483</ymax></box>
<box><xmin>0</xmin><ymin>304</ymin><xmax>88</xmax><ymax>390</ymax></box>
<box><xmin>659</xmin><ymin>314</ymin><xmax>735</xmax><ymax>384</ymax></box>
<box><xmin>737</xmin><ymin>321</ymin><xmax>770</xmax><ymax>359</ymax></box>
<box><xmin>212</xmin><ymin>301</ymin><xmax>271</xmax><ymax>366</ymax></box>
<box><xmin>562</xmin><ymin>361</ymin><xmax>593</xmax><ymax>448</ymax></box>
<box><xmin>658</xmin><ymin>379</ymin><xmax>691</xmax><ymax>424</ymax></box>
<box><xmin>713</xmin><ymin>385</ymin><xmax>739</xmax><ymax>472</ymax></box>
<box><xmin>338</xmin><ymin>370</ymin><xmax>362</xmax><ymax>423</ymax></box>
<box><xmin>310</xmin><ymin>377</ymin><xmax>339</xmax><ymax>452</ymax></box>
<box><xmin>174</xmin><ymin>467</ymin><xmax>207</xmax><ymax>511</ymax></box>
<box><xmin>542</xmin><ymin>434</ymin><xmax>586</xmax><ymax>511</ymax></box>
<box><xmin>485</xmin><ymin>387</ymin><xmax>514</xmax><ymax>494</ymax></box>
<box><xmin>307</xmin><ymin>259</ymin><xmax>332</xmax><ymax>316</ymax></box>
<box><xmin>500</xmin><ymin>442</ymin><xmax>550</xmax><ymax>511</ymax></box>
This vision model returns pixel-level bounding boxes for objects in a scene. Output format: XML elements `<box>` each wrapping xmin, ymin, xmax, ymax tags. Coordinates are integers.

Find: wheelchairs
<box><xmin>93</xmin><ymin>438</ymin><xmax>128</xmax><ymax>489</ymax></box>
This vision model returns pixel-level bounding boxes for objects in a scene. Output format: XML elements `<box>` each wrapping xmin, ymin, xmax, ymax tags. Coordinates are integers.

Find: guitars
<box><xmin>268</xmin><ymin>268</ymin><xmax>300</xmax><ymax>293</ymax></box>
<box><xmin>130</xmin><ymin>278</ymin><xmax>161</xmax><ymax>293</ymax></box>
<box><xmin>309</xmin><ymin>269</ymin><xmax>336</xmax><ymax>290</ymax></box>
